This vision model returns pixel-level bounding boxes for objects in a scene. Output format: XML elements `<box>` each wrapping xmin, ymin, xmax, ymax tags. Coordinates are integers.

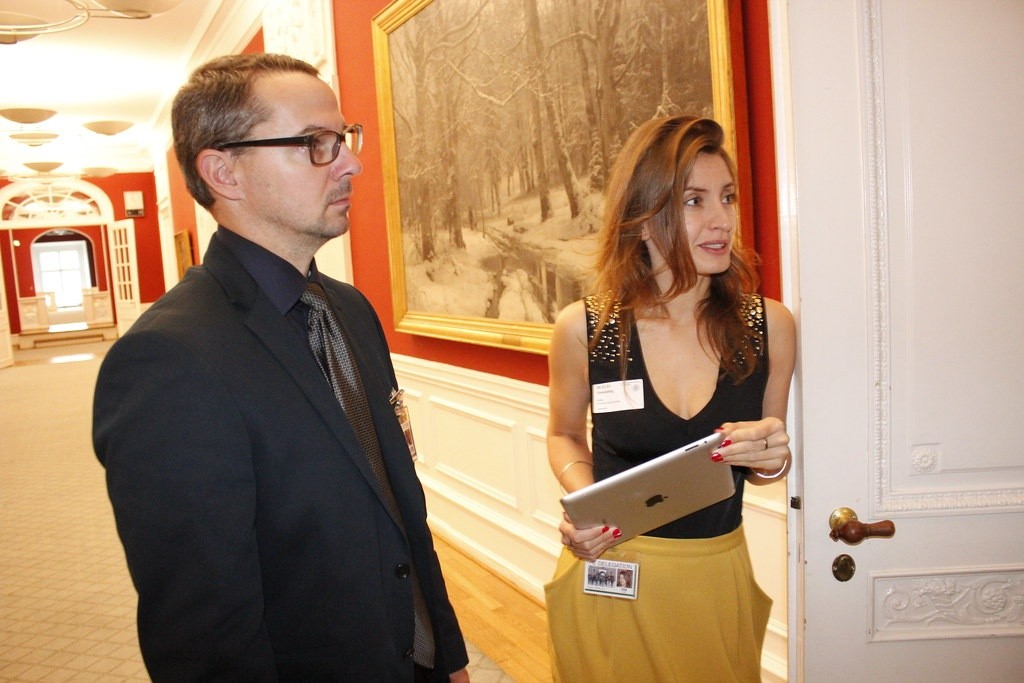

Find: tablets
<box><xmin>560</xmin><ymin>432</ymin><xmax>734</xmax><ymax>552</ymax></box>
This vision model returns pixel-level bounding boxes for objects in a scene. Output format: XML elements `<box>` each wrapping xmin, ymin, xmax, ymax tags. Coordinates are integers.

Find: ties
<box><xmin>298</xmin><ymin>280</ymin><xmax>437</xmax><ymax>669</ymax></box>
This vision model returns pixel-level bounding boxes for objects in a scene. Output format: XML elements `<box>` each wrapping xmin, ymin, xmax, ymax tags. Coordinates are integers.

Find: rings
<box><xmin>762</xmin><ymin>437</ymin><xmax>768</xmax><ymax>449</ymax></box>
<box><xmin>570</xmin><ymin>540</ymin><xmax>572</xmax><ymax>546</ymax></box>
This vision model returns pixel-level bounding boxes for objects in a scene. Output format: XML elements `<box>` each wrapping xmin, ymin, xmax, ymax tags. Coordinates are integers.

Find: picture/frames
<box><xmin>370</xmin><ymin>0</ymin><xmax>739</xmax><ymax>356</ymax></box>
<box><xmin>174</xmin><ymin>228</ymin><xmax>191</xmax><ymax>280</ymax></box>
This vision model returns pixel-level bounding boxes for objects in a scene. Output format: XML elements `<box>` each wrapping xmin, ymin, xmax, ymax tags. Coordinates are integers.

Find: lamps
<box><xmin>0</xmin><ymin>109</ymin><xmax>133</xmax><ymax>171</ymax></box>
<box><xmin>0</xmin><ymin>0</ymin><xmax>177</xmax><ymax>45</ymax></box>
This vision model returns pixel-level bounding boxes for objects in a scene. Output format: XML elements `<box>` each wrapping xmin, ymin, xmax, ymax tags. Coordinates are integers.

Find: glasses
<box><xmin>216</xmin><ymin>122</ymin><xmax>364</xmax><ymax>166</ymax></box>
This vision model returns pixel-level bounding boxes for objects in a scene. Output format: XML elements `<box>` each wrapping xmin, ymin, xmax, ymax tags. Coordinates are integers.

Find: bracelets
<box><xmin>751</xmin><ymin>459</ymin><xmax>788</xmax><ymax>479</ymax></box>
<box><xmin>559</xmin><ymin>461</ymin><xmax>594</xmax><ymax>496</ymax></box>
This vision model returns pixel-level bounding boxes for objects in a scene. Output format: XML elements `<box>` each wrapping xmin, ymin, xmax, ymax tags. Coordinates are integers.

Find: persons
<box><xmin>92</xmin><ymin>54</ymin><xmax>470</xmax><ymax>683</ymax></box>
<box><xmin>588</xmin><ymin>570</ymin><xmax>631</xmax><ymax>588</ymax></box>
<box><xmin>544</xmin><ymin>114</ymin><xmax>797</xmax><ymax>683</ymax></box>
